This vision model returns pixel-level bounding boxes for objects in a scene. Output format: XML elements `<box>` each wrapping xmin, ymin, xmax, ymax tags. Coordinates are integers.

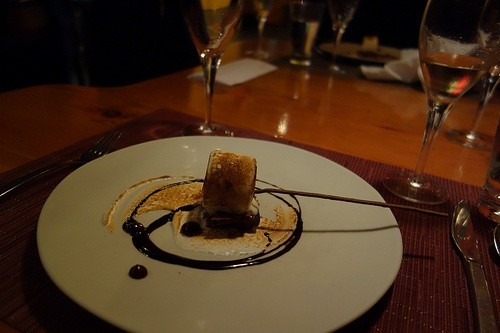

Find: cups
<box><xmin>477</xmin><ymin>118</ymin><xmax>500</xmax><ymax>224</ymax></box>
<box><xmin>286</xmin><ymin>0</ymin><xmax>325</xmax><ymax>67</ymax></box>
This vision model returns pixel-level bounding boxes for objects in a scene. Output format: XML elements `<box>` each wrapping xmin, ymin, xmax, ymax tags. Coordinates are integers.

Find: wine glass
<box><xmin>443</xmin><ymin>0</ymin><xmax>500</xmax><ymax>151</ymax></box>
<box><xmin>181</xmin><ymin>0</ymin><xmax>247</xmax><ymax>137</ymax></box>
<box><xmin>326</xmin><ymin>0</ymin><xmax>359</xmax><ymax>75</ymax></box>
<box><xmin>241</xmin><ymin>0</ymin><xmax>279</xmax><ymax>60</ymax></box>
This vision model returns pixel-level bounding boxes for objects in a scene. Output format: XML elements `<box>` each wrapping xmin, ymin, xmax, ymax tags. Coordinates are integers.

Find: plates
<box><xmin>319</xmin><ymin>42</ymin><xmax>401</xmax><ymax>63</ymax></box>
<box><xmin>36</xmin><ymin>136</ymin><xmax>403</xmax><ymax>333</ymax></box>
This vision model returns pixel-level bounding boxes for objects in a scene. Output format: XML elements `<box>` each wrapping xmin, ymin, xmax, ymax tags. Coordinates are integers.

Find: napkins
<box><xmin>189</xmin><ymin>59</ymin><xmax>277</xmax><ymax>85</ymax></box>
<box><xmin>361</xmin><ymin>37</ymin><xmax>477</xmax><ymax>90</ymax></box>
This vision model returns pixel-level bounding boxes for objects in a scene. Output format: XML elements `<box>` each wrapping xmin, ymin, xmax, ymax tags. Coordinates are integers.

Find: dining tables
<box><xmin>0</xmin><ymin>32</ymin><xmax>499</xmax><ymax>333</ymax></box>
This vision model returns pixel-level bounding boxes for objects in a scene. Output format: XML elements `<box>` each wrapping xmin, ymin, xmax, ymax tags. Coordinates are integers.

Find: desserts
<box><xmin>202</xmin><ymin>150</ymin><xmax>257</xmax><ymax>215</ymax></box>
<box><xmin>362</xmin><ymin>36</ymin><xmax>378</xmax><ymax>51</ymax></box>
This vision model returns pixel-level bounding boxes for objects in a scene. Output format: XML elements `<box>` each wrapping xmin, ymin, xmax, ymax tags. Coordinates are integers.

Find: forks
<box><xmin>0</xmin><ymin>130</ymin><xmax>122</xmax><ymax>197</ymax></box>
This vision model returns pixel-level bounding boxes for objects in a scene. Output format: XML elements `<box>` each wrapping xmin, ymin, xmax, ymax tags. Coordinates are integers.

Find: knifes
<box><xmin>451</xmin><ymin>199</ymin><xmax>497</xmax><ymax>333</ymax></box>
